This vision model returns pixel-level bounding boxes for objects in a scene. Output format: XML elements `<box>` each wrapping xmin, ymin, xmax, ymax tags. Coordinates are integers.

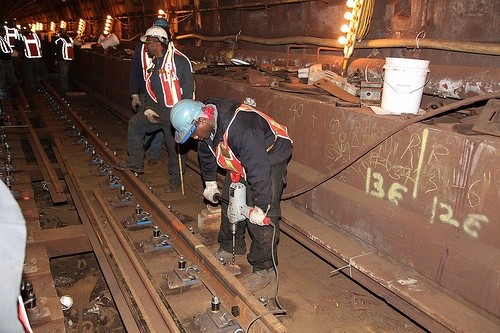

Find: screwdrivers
<box><xmin>335</xmin><ymin>102</ymin><xmax>380</xmax><ymax>107</ymax></box>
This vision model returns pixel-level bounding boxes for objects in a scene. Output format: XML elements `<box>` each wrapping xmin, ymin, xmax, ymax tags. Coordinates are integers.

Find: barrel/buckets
<box><xmin>381</xmin><ymin>57</ymin><xmax>430</xmax><ymax>115</ymax></box>
<box><xmin>101</xmin><ymin>33</ymin><xmax>120</xmax><ymax>49</ymax></box>
<box><xmin>97</xmin><ymin>34</ymin><xmax>105</xmax><ymax>44</ymax></box>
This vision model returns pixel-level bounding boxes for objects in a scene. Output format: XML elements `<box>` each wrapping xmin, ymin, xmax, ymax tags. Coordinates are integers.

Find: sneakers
<box><xmin>239</xmin><ymin>266</ymin><xmax>276</xmax><ymax>292</ymax></box>
<box><xmin>163</xmin><ymin>183</ymin><xmax>182</xmax><ymax>193</ymax></box>
<box><xmin>213</xmin><ymin>248</ymin><xmax>246</xmax><ymax>262</ymax></box>
<box><xmin>115</xmin><ymin>162</ymin><xmax>143</xmax><ymax>174</ymax></box>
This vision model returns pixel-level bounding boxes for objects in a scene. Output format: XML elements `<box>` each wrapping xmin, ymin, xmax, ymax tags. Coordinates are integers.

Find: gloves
<box><xmin>203</xmin><ymin>181</ymin><xmax>220</xmax><ymax>204</ymax></box>
<box><xmin>131</xmin><ymin>94</ymin><xmax>142</xmax><ymax>113</ymax></box>
<box><xmin>144</xmin><ymin>109</ymin><xmax>159</xmax><ymax>123</ymax></box>
<box><xmin>250</xmin><ymin>204</ymin><xmax>270</xmax><ymax>226</ymax></box>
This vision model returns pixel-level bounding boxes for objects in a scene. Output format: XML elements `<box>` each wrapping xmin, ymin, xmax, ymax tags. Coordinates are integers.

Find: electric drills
<box><xmin>212</xmin><ymin>172</ymin><xmax>271</xmax><ymax>266</ymax></box>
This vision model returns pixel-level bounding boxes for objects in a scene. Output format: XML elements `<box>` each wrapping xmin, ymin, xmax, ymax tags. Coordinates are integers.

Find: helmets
<box><xmin>152</xmin><ymin>18</ymin><xmax>168</xmax><ymax>28</ymax></box>
<box><xmin>169</xmin><ymin>99</ymin><xmax>203</xmax><ymax>144</ymax></box>
<box><xmin>140</xmin><ymin>26</ymin><xmax>169</xmax><ymax>43</ymax></box>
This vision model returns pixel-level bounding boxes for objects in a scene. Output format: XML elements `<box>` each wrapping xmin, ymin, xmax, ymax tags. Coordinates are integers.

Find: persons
<box><xmin>0</xmin><ymin>20</ymin><xmax>74</xmax><ymax>92</ymax></box>
<box><xmin>0</xmin><ymin>179</ymin><xmax>33</xmax><ymax>333</ymax></box>
<box><xmin>169</xmin><ymin>98</ymin><xmax>294</xmax><ymax>293</ymax></box>
<box><xmin>111</xmin><ymin>18</ymin><xmax>195</xmax><ymax>189</ymax></box>
